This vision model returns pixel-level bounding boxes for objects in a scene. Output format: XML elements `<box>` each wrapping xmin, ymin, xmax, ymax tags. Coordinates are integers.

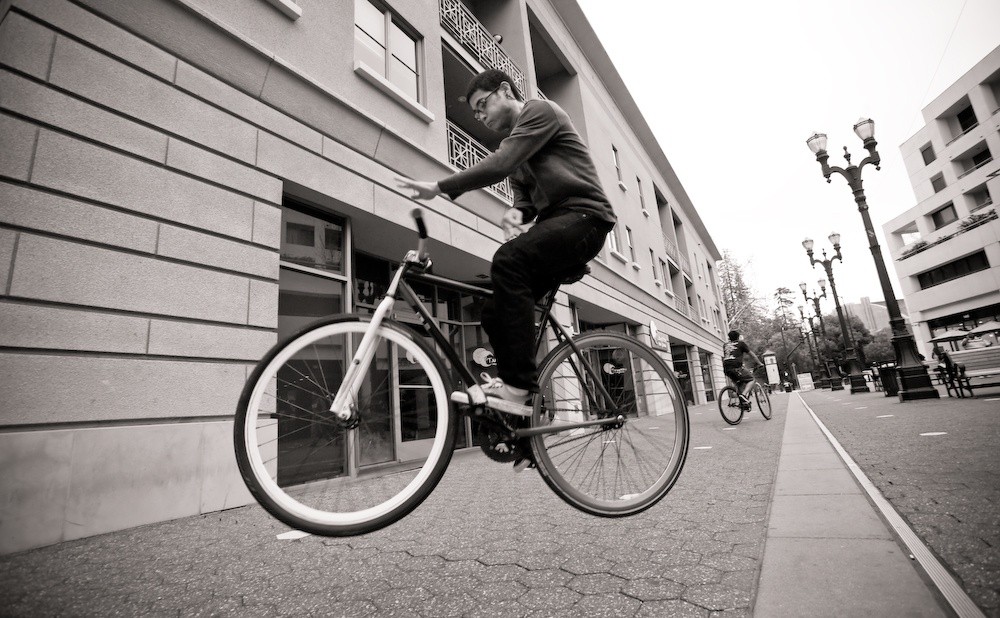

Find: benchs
<box><xmin>921</xmin><ymin>346</ymin><xmax>1000</xmax><ymax>398</ymax></box>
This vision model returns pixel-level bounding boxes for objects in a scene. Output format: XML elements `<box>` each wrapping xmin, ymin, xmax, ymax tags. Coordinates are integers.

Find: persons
<box><xmin>962</xmin><ymin>332</ymin><xmax>993</xmax><ymax>379</ymax></box>
<box><xmin>723</xmin><ymin>331</ymin><xmax>763</xmax><ymax>404</ymax></box>
<box><xmin>394</xmin><ymin>69</ymin><xmax>618</xmax><ymax>414</ymax></box>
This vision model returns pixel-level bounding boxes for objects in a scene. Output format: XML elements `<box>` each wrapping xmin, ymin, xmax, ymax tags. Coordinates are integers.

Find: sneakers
<box><xmin>451</xmin><ymin>373</ymin><xmax>534</xmax><ymax>416</ymax></box>
<box><xmin>739</xmin><ymin>395</ymin><xmax>749</xmax><ymax>402</ymax></box>
<box><xmin>513</xmin><ymin>457</ymin><xmax>531</xmax><ymax>474</ymax></box>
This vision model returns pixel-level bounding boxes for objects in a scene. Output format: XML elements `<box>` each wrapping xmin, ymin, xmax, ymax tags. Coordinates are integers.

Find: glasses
<box><xmin>473</xmin><ymin>86</ymin><xmax>500</xmax><ymax>121</ymax></box>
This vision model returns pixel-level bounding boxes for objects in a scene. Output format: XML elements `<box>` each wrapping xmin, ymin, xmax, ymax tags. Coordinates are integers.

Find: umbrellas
<box><xmin>927</xmin><ymin>329</ymin><xmax>969</xmax><ymax>352</ymax></box>
<box><xmin>962</xmin><ymin>320</ymin><xmax>1000</xmax><ymax>346</ymax></box>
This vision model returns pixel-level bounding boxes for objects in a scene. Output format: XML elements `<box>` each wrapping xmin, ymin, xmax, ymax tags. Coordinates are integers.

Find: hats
<box><xmin>728</xmin><ymin>331</ymin><xmax>740</xmax><ymax>341</ymax></box>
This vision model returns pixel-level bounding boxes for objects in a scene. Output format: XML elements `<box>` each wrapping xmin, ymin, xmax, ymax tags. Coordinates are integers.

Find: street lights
<box><xmin>798</xmin><ymin>303</ymin><xmax>832</xmax><ymax>389</ymax></box>
<box><xmin>800</xmin><ymin>231</ymin><xmax>870</xmax><ymax>395</ymax></box>
<box><xmin>805</xmin><ymin>116</ymin><xmax>941</xmax><ymax>402</ymax></box>
<box><xmin>798</xmin><ymin>278</ymin><xmax>845</xmax><ymax>391</ymax></box>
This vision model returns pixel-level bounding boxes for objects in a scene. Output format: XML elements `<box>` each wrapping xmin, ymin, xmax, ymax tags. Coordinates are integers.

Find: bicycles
<box><xmin>230</xmin><ymin>208</ymin><xmax>693</xmax><ymax>541</ymax></box>
<box><xmin>717</xmin><ymin>364</ymin><xmax>773</xmax><ymax>426</ymax></box>
